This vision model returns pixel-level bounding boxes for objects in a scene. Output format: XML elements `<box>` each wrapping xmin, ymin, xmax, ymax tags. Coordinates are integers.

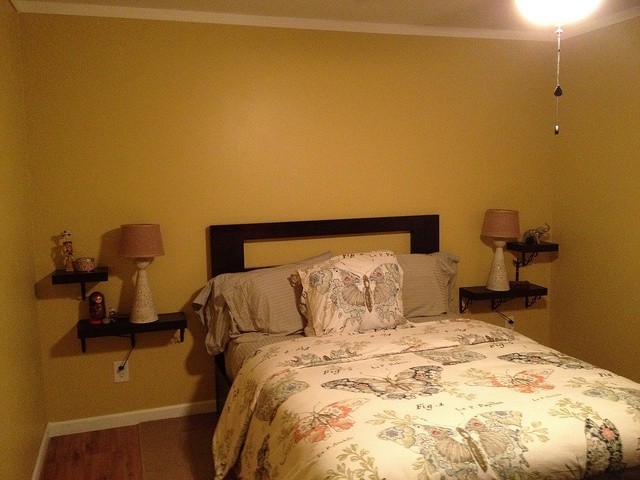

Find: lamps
<box><xmin>480</xmin><ymin>208</ymin><xmax>521</xmax><ymax>292</ymax></box>
<box><xmin>119</xmin><ymin>223</ymin><xmax>166</xmax><ymax>324</ymax></box>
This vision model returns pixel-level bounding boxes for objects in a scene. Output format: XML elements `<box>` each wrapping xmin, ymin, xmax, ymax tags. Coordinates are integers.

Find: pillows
<box><xmin>396</xmin><ymin>250</ymin><xmax>461</xmax><ymax>324</ymax></box>
<box><xmin>220</xmin><ymin>249</ymin><xmax>335</xmax><ymax>342</ymax></box>
<box><xmin>288</xmin><ymin>249</ymin><xmax>415</xmax><ymax>337</ymax></box>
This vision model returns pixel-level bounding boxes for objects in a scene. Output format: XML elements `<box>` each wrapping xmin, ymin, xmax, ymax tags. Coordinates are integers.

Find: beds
<box><xmin>208</xmin><ymin>214</ymin><xmax>640</xmax><ymax>480</ymax></box>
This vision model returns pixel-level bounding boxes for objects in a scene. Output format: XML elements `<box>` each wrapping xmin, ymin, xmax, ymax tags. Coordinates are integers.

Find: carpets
<box><xmin>138</xmin><ymin>411</ymin><xmax>217</xmax><ymax>480</ymax></box>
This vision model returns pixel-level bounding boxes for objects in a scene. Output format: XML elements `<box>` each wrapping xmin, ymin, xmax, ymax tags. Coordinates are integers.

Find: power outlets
<box><xmin>505</xmin><ymin>316</ymin><xmax>515</xmax><ymax>331</ymax></box>
<box><xmin>112</xmin><ymin>360</ymin><xmax>130</xmax><ymax>383</ymax></box>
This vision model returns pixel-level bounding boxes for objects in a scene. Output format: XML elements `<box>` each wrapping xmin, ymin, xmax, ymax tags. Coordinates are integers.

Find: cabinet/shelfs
<box><xmin>459</xmin><ymin>241</ymin><xmax>559</xmax><ymax>314</ymax></box>
<box><xmin>51</xmin><ymin>266</ymin><xmax>188</xmax><ymax>353</ymax></box>
